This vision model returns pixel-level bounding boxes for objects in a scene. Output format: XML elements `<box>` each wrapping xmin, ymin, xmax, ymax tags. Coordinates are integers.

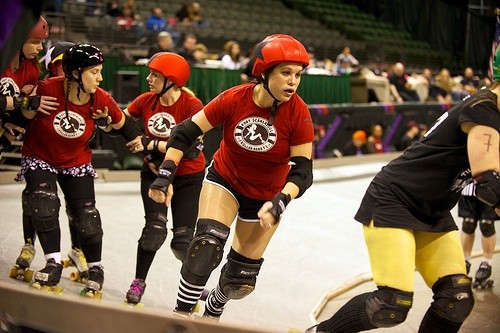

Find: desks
<box><xmin>144</xmin><ymin>65</ymin><xmax>353</xmax><ymax>113</ymax></box>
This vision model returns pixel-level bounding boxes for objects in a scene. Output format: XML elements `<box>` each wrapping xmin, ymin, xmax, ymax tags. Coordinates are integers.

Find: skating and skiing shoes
<box><xmin>194</xmin><ymin>289</ymin><xmax>209</xmax><ymax>312</ymax></box>
<box><xmin>80</xmin><ymin>266</ymin><xmax>104</xmax><ymax>301</ymax></box>
<box><xmin>471</xmin><ymin>262</ymin><xmax>494</xmax><ymax>291</ymax></box>
<box><xmin>122</xmin><ymin>280</ymin><xmax>145</xmax><ymax>309</ymax></box>
<box><xmin>32</xmin><ymin>258</ymin><xmax>64</xmax><ymax>294</ymax></box>
<box><xmin>10</xmin><ymin>244</ymin><xmax>36</xmax><ymax>282</ymax></box>
<box><xmin>62</xmin><ymin>248</ymin><xmax>89</xmax><ymax>284</ymax></box>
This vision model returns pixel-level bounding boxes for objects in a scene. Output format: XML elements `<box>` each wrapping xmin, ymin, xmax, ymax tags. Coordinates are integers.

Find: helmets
<box><xmin>27</xmin><ymin>16</ymin><xmax>49</xmax><ymax>39</ymax></box>
<box><xmin>246</xmin><ymin>35</ymin><xmax>310</xmax><ymax>80</ymax></box>
<box><xmin>148</xmin><ymin>54</ymin><xmax>191</xmax><ymax>86</ymax></box>
<box><xmin>63</xmin><ymin>45</ymin><xmax>104</xmax><ymax>73</ymax></box>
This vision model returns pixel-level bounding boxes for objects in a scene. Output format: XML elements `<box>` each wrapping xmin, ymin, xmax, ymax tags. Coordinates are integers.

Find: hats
<box><xmin>46</xmin><ymin>41</ymin><xmax>73</xmax><ymax>71</ymax></box>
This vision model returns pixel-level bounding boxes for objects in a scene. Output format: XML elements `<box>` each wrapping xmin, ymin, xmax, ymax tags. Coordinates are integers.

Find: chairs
<box><xmin>67</xmin><ymin>0</ymin><xmax>445</xmax><ymax>64</ymax></box>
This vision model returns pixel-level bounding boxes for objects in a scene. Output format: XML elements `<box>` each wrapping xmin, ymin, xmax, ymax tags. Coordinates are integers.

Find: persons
<box><xmin>84</xmin><ymin>0</ymin><xmax>491</xmax><ymax>104</ymax></box>
<box><xmin>311</xmin><ymin>46</ymin><xmax>500</xmax><ymax>333</ymax></box>
<box><xmin>148</xmin><ymin>33</ymin><xmax>315</xmax><ymax>324</ymax></box>
<box><xmin>0</xmin><ymin>15</ymin><xmax>210</xmax><ymax>316</ymax></box>
<box><xmin>456</xmin><ymin>178</ymin><xmax>500</xmax><ymax>288</ymax></box>
<box><xmin>314</xmin><ymin>117</ymin><xmax>432</xmax><ymax>158</ymax></box>
